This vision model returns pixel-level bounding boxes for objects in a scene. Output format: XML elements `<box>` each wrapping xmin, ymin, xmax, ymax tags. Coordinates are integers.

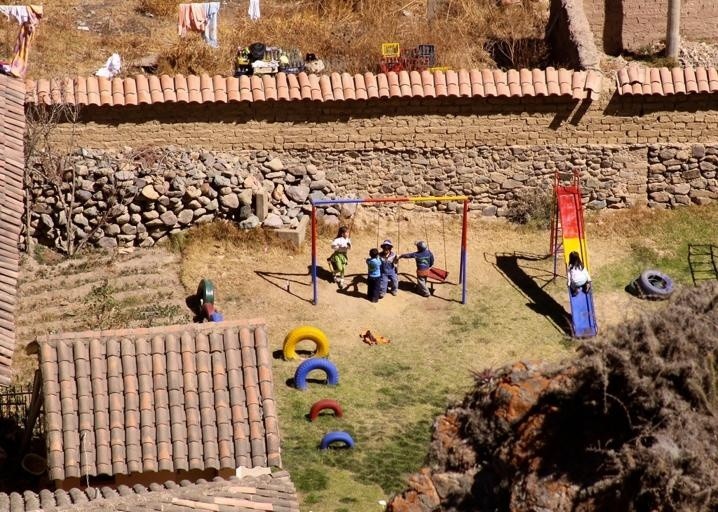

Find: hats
<box><xmin>381</xmin><ymin>239</ymin><xmax>393</xmax><ymax>248</ymax></box>
<box><xmin>414</xmin><ymin>240</ymin><xmax>426</xmax><ymax>249</ymax></box>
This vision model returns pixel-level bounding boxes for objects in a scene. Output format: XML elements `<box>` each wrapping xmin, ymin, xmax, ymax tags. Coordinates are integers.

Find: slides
<box><xmin>557</xmin><ymin>194</ymin><xmax>597</xmax><ymax>340</ymax></box>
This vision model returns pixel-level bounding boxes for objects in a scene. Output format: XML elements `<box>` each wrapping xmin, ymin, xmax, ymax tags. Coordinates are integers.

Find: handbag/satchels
<box><xmin>234</xmin><ymin>42</ymin><xmax>324</xmax><ymax>75</ymax></box>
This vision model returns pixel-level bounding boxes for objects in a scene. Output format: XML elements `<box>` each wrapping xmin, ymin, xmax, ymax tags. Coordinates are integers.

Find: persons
<box><xmin>566</xmin><ymin>250</ymin><xmax>592</xmax><ymax>297</ymax></box>
<box><xmin>397</xmin><ymin>241</ymin><xmax>435</xmax><ymax>297</ymax></box>
<box><xmin>379</xmin><ymin>240</ymin><xmax>397</xmax><ymax>298</ymax></box>
<box><xmin>330</xmin><ymin>225</ymin><xmax>352</xmax><ymax>288</ymax></box>
<box><xmin>367</xmin><ymin>249</ymin><xmax>382</xmax><ymax>303</ymax></box>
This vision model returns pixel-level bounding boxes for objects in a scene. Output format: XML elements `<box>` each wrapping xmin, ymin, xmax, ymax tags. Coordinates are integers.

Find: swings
<box><xmin>377</xmin><ymin>200</ymin><xmax>401</xmax><ymax>274</ymax></box>
<box><xmin>327</xmin><ymin>202</ymin><xmax>358</xmax><ymax>266</ymax></box>
<box><xmin>422</xmin><ymin>201</ymin><xmax>450</xmax><ymax>281</ymax></box>
